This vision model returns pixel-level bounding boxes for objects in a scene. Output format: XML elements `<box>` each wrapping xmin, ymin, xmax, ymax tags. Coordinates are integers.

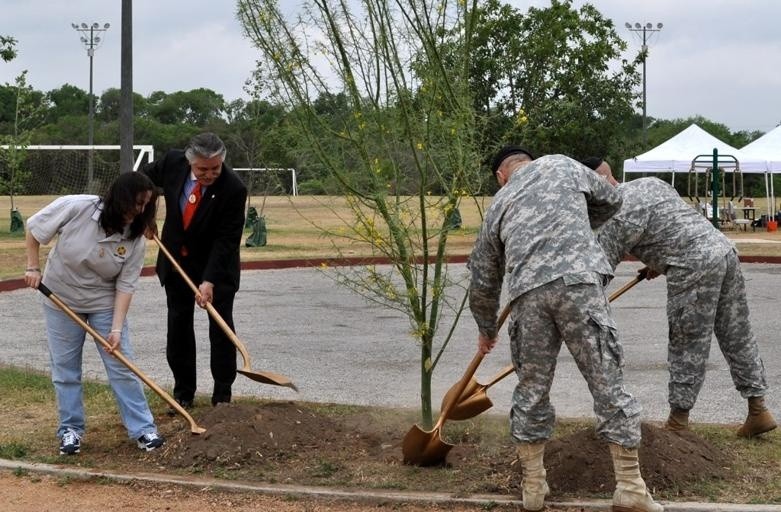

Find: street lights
<box><xmin>625</xmin><ymin>23</ymin><xmax>664</xmax><ymax>178</ymax></box>
<box><xmin>71</xmin><ymin>23</ymin><xmax>111</xmax><ymax>195</ymax></box>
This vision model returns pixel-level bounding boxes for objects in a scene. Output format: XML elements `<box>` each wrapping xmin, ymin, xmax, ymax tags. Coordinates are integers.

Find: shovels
<box><xmin>153</xmin><ymin>233</ymin><xmax>293</xmax><ymax>387</ymax></box>
<box><xmin>441</xmin><ymin>267</ymin><xmax>651</xmax><ymax>421</ymax></box>
<box><xmin>37</xmin><ymin>281</ymin><xmax>207</xmax><ymax>436</ymax></box>
<box><xmin>401</xmin><ymin>300</ymin><xmax>514</xmax><ymax>468</ymax></box>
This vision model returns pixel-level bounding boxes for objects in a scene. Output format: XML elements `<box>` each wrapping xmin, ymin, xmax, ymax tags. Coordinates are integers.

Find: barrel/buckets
<box><xmin>766</xmin><ymin>221</ymin><xmax>778</xmax><ymax>233</ymax></box>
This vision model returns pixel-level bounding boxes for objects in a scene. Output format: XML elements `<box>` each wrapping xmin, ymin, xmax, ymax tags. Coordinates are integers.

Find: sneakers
<box><xmin>138</xmin><ymin>433</ymin><xmax>167</xmax><ymax>452</ymax></box>
<box><xmin>60</xmin><ymin>428</ymin><xmax>82</xmax><ymax>455</ymax></box>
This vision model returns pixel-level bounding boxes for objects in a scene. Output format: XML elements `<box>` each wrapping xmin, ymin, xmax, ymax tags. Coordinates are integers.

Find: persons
<box><xmin>577</xmin><ymin>153</ymin><xmax>778</xmax><ymax>437</ymax></box>
<box><xmin>138</xmin><ymin>133</ymin><xmax>253</xmax><ymax>415</ymax></box>
<box><xmin>24</xmin><ymin>172</ymin><xmax>166</xmax><ymax>454</ymax></box>
<box><xmin>469</xmin><ymin>149</ymin><xmax>667</xmax><ymax>511</ymax></box>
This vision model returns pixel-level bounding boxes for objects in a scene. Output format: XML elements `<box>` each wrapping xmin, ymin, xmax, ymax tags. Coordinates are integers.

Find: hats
<box><xmin>492</xmin><ymin>146</ymin><xmax>533</xmax><ymax>174</ymax></box>
<box><xmin>580</xmin><ymin>157</ymin><xmax>603</xmax><ymax>171</ymax></box>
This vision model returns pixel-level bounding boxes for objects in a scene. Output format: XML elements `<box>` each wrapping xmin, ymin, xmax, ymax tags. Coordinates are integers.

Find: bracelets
<box><xmin>27</xmin><ymin>267</ymin><xmax>40</xmax><ymax>273</ymax></box>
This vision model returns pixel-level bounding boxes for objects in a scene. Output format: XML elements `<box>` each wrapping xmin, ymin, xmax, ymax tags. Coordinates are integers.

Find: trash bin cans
<box><xmin>768</xmin><ymin>220</ymin><xmax>778</xmax><ymax>232</ymax></box>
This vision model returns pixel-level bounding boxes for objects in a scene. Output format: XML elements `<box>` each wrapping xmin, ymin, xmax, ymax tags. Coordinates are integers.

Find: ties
<box><xmin>179</xmin><ymin>181</ymin><xmax>202</xmax><ymax>256</ymax></box>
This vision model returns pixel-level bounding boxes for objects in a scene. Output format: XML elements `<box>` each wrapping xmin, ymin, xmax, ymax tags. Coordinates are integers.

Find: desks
<box><xmin>737</xmin><ymin>206</ymin><xmax>759</xmax><ymax>233</ymax></box>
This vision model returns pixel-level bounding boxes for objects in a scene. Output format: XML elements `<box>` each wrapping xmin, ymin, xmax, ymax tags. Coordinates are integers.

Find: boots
<box><xmin>737</xmin><ymin>396</ymin><xmax>778</xmax><ymax>437</ymax></box>
<box><xmin>663</xmin><ymin>406</ymin><xmax>690</xmax><ymax>432</ymax></box>
<box><xmin>513</xmin><ymin>443</ymin><xmax>551</xmax><ymax>511</ymax></box>
<box><xmin>609</xmin><ymin>443</ymin><xmax>664</xmax><ymax>512</ymax></box>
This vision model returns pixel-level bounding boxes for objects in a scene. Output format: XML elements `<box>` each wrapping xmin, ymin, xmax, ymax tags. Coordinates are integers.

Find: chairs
<box><xmin>728</xmin><ymin>201</ymin><xmax>749</xmax><ymax>234</ymax></box>
